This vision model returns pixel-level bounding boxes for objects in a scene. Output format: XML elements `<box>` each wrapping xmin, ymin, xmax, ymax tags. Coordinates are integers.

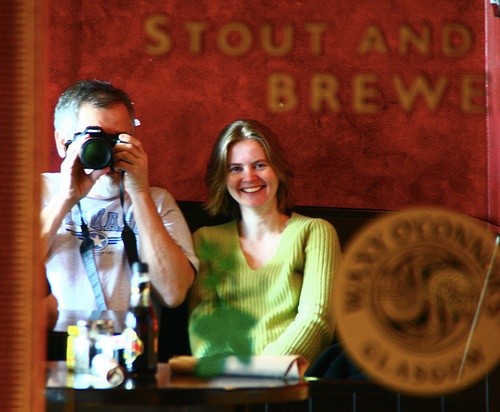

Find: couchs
<box><xmin>157</xmin><ymin>200</ymin><xmax>486</xmax><ymax>411</ymax></box>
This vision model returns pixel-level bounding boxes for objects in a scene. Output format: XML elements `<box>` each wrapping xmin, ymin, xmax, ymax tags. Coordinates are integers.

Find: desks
<box><xmin>44</xmin><ymin>360</ymin><xmax>325</xmax><ymax>412</ymax></box>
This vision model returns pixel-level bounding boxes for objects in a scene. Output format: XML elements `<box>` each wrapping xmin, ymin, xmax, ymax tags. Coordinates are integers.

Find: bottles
<box><xmin>124</xmin><ymin>262</ymin><xmax>159</xmax><ymax>375</ymax></box>
<box><xmin>67</xmin><ymin>326</ymin><xmax>77</xmax><ymax>371</ymax></box>
<box><xmin>95</xmin><ymin>320</ymin><xmax>119</xmax><ymax>365</ymax></box>
<box><xmin>74</xmin><ymin>320</ymin><xmax>95</xmax><ymax>373</ymax></box>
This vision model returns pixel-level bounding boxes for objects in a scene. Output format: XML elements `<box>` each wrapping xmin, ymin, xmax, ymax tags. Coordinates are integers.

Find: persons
<box><xmin>39</xmin><ymin>81</ymin><xmax>200</xmax><ymax>361</ymax></box>
<box><xmin>188</xmin><ymin>119</ymin><xmax>341</xmax><ymax>364</ymax></box>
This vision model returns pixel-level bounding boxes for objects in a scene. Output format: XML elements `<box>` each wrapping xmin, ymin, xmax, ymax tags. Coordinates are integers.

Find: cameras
<box><xmin>73</xmin><ymin>125</ymin><xmax>122</xmax><ymax>170</ymax></box>
